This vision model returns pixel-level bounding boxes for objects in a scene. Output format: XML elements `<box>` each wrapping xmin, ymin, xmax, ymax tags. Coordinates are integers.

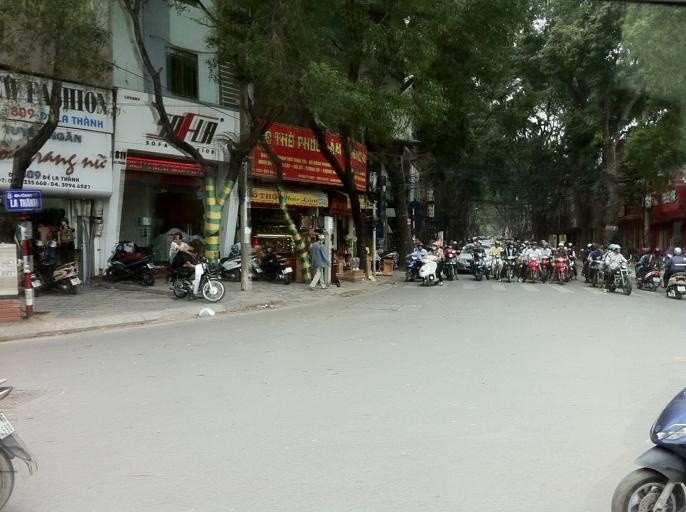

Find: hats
<box><xmin>317</xmin><ymin>234</ymin><xmax>325</xmax><ymax>238</ymax></box>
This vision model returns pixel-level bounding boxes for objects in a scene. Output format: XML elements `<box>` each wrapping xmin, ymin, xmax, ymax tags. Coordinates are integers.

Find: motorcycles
<box><xmin>31</xmin><ymin>260</ymin><xmax>82</xmax><ymax>297</ymax></box>
<box><xmin>105</xmin><ymin>242</ymin><xmax>293</xmax><ymax>302</ymax></box>
<box><xmin>406</xmin><ymin>251</ymin><xmax>686</xmax><ymax>299</ymax></box>
<box><xmin>0</xmin><ymin>378</ymin><xmax>39</xmax><ymax>512</ymax></box>
<box><xmin>611</xmin><ymin>387</ymin><xmax>686</xmax><ymax>512</ymax></box>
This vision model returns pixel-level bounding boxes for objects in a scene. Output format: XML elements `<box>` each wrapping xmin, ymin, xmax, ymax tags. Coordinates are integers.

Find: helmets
<box><xmin>418</xmin><ymin>239</ymin><xmax>682</xmax><ymax>255</ymax></box>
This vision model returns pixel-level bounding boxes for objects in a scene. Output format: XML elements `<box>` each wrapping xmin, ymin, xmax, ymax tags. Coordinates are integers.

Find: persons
<box><xmin>169</xmin><ymin>232</ymin><xmax>197</xmax><ymax>270</ymax></box>
<box><xmin>404</xmin><ymin>240</ymin><xmax>686</xmax><ymax>299</ymax></box>
<box><xmin>37</xmin><ymin>221</ymin><xmax>52</xmax><ymax>242</ymax></box>
<box><xmin>309</xmin><ymin>233</ymin><xmax>330</xmax><ymax>290</ymax></box>
<box><xmin>57</xmin><ymin>216</ymin><xmax>75</xmax><ymax>262</ymax></box>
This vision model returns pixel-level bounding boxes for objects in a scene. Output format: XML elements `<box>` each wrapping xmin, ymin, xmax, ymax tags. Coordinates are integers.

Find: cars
<box><xmin>456</xmin><ymin>242</ymin><xmax>491</xmax><ymax>274</ymax></box>
